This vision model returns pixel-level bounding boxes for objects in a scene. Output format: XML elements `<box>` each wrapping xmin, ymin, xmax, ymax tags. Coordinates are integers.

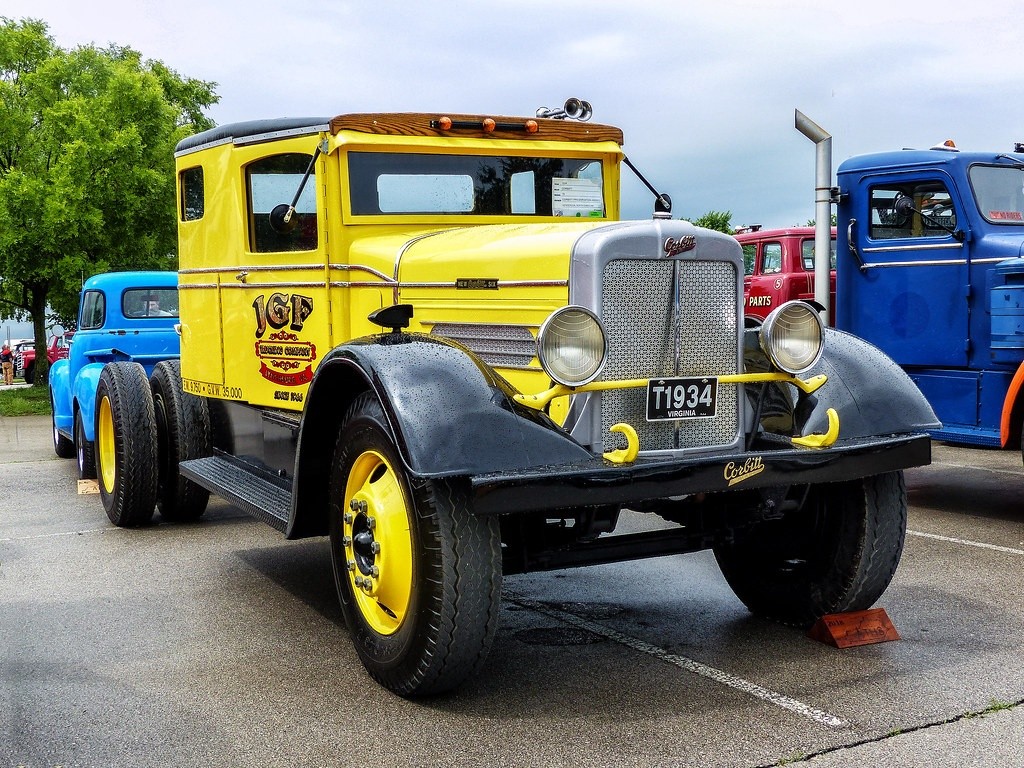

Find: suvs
<box><xmin>16</xmin><ymin>330</ymin><xmax>76</xmax><ymax>385</ymax></box>
<box><xmin>48</xmin><ymin>270</ymin><xmax>180</xmax><ymax>478</ymax></box>
<box><xmin>733</xmin><ymin>225</ymin><xmax>836</xmax><ymax>330</ymax></box>
<box><xmin>93</xmin><ymin>99</ymin><xmax>943</xmax><ymax>701</ymax></box>
<box><xmin>793</xmin><ymin>109</ymin><xmax>1024</xmax><ymax>447</ymax></box>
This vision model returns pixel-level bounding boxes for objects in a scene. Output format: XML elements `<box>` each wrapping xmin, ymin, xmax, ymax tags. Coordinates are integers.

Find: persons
<box><xmin>140</xmin><ymin>295</ymin><xmax>173</xmax><ymax>317</ymax></box>
<box><xmin>0</xmin><ymin>344</ymin><xmax>16</xmax><ymax>385</ymax></box>
